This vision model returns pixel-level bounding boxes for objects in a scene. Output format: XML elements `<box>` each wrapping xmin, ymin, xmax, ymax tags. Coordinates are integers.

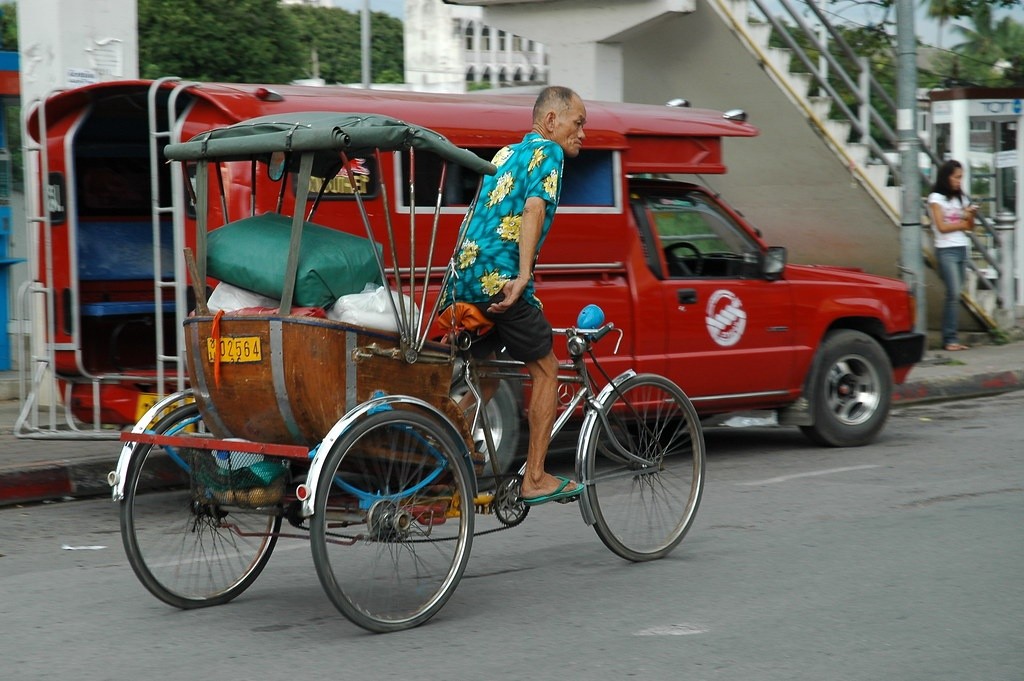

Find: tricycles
<box><xmin>107</xmin><ymin>111</ymin><xmax>706</xmax><ymax>635</ymax></box>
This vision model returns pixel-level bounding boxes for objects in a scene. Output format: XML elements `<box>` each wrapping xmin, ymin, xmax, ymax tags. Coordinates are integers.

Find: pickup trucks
<box><xmin>13</xmin><ymin>79</ymin><xmax>927</xmax><ymax>517</ymax></box>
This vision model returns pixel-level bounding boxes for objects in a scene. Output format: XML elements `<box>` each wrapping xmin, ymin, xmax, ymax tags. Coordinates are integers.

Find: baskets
<box><xmin>190</xmin><ymin>446</ymin><xmax>289</xmax><ymax>510</ymax></box>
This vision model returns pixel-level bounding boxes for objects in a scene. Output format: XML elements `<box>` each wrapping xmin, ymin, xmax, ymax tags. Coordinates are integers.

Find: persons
<box><xmin>927</xmin><ymin>161</ymin><xmax>978</xmax><ymax>351</ymax></box>
<box><xmin>439</xmin><ymin>86</ymin><xmax>586</xmax><ymax>505</ymax></box>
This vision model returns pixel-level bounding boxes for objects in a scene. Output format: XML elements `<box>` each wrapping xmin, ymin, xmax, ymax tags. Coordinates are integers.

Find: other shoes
<box><xmin>945</xmin><ymin>343</ymin><xmax>968</xmax><ymax>350</ymax></box>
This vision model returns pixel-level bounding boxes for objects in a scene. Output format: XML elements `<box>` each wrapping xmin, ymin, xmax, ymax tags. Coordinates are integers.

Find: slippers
<box><xmin>523</xmin><ymin>476</ymin><xmax>584</xmax><ymax>506</ymax></box>
